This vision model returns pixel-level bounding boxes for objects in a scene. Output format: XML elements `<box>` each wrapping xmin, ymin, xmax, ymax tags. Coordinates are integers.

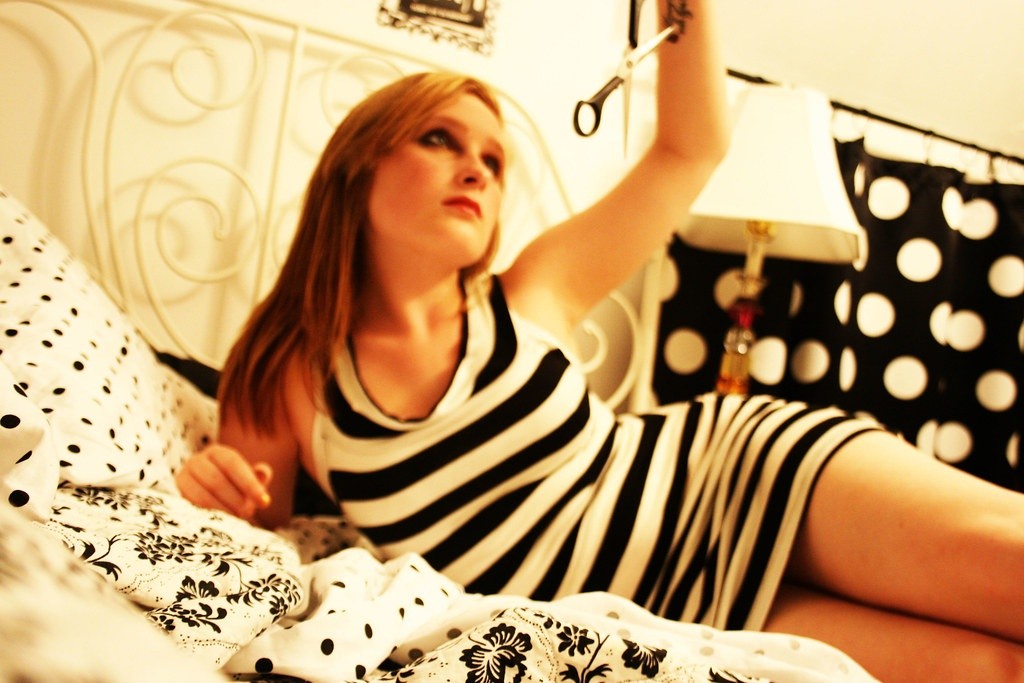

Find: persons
<box><xmin>182</xmin><ymin>0</ymin><xmax>1024</xmax><ymax>682</ymax></box>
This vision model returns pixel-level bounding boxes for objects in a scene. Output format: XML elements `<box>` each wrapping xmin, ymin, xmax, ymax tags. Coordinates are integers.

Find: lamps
<box><xmin>674</xmin><ymin>85</ymin><xmax>861</xmax><ymax>400</ymax></box>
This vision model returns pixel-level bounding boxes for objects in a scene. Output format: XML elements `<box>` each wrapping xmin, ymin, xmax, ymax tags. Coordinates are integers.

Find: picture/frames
<box><xmin>375</xmin><ymin>0</ymin><xmax>502</xmax><ymax>56</ymax></box>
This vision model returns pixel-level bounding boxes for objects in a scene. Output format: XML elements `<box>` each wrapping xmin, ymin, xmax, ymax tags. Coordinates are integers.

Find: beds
<box><xmin>0</xmin><ymin>0</ymin><xmax>889</xmax><ymax>683</ymax></box>
